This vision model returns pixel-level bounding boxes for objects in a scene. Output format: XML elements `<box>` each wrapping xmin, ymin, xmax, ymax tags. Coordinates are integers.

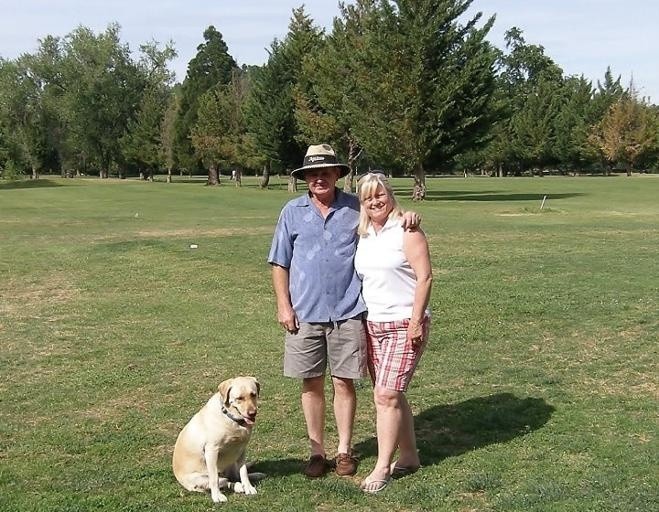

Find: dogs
<box><xmin>171</xmin><ymin>375</ymin><xmax>267</xmax><ymax>504</ymax></box>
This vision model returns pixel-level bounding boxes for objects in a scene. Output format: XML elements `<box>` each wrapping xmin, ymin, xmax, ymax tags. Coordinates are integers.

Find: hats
<box><xmin>290</xmin><ymin>144</ymin><xmax>351</xmax><ymax>180</ymax></box>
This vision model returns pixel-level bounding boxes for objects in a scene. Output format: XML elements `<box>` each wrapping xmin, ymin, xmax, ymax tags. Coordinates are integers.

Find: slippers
<box><xmin>358</xmin><ymin>465</ymin><xmax>407</xmax><ymax>494</ymax></box>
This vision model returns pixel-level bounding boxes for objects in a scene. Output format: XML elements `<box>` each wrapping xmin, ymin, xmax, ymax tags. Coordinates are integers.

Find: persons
<box><xmin>354</xmin><ymin>170</ymin><xmax>433</xmax><ymax>492</ymax></box>
<box><xmin>411</xmin><ymin>178</ymin><xmax>426</xmax><ymax>201</ymax></box>
<box><xmin>267</xmin><ymin>144</ymin><xmax>421</xmax><ymax>478</ymax></box>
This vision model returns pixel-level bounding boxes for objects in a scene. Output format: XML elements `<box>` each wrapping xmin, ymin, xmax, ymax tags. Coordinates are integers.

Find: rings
<box><xmin>413</xmin><ymin>340</ymin><xmax>417</xmax><ymax>342</ymax></box>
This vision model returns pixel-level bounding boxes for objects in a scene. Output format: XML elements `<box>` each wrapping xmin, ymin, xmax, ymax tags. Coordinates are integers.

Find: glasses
<box><xmin>357</xmin><ymin>170</ymin><xmax>387</xmax><ymax>181</ymax></box>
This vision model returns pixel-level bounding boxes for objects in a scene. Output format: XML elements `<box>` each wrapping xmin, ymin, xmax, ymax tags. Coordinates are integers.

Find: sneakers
<box><xmin>303</xmin><ymin>452</ymin><xmax>358</xmax><ymax>478</ymax></box>
